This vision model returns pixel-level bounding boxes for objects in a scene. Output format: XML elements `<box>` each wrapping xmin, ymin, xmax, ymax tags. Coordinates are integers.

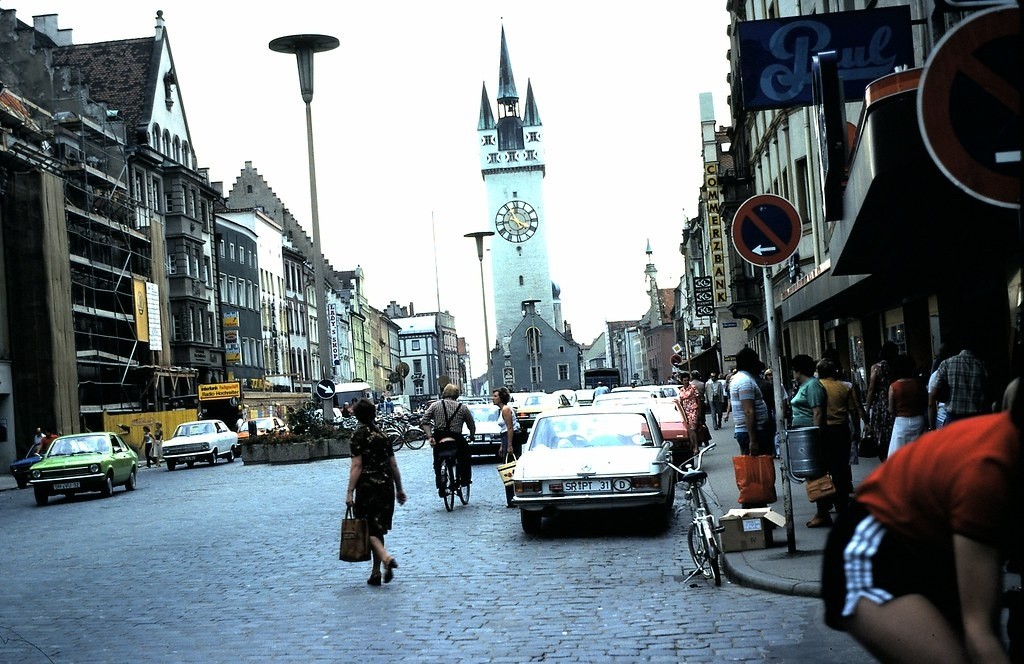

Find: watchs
<box><xmin>928</xmin><ymin>428</ymin><xmax>936</xmax><ymax>431</ymax></box>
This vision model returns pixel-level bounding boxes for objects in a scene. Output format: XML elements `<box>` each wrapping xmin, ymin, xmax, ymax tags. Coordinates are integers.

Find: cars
<box><xmin>506</xmin><ymin>383</ymin><xmax>693</xmax><ymax>477</ymax></box>
<box><xmin>161</xmin><ymin>419</ymin><xmax>238</xmax><ymax>471</ymax></box>
<box><xmin>235</xmin><ymin>416</ymin><xmax>291</xmax><ymax>444</ymax></box>
<box><xmin>509</xmin><ymin>406</ymin><xmax>677</xmax><ymax>535</ymax></box>
<box><xmin>460</xmin><ymin>404</ymin><xmax>522</xmax><ymax>463</ymax></box>
<box><xmin>28</xmin><ymin>432</ymin><xmax>139</xmax><ymax>507</ymax></box>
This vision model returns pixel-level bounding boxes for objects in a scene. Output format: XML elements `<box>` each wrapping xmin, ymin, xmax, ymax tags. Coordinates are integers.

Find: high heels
<box><xmin>367</xmin><ymin>571</ymin><xmax>382</xmax><ymax>586</ymax></box>
<box><xmin>383</xmin><ymin>556</ymin><xmax>398</xmax><ymax>583</ymax></box>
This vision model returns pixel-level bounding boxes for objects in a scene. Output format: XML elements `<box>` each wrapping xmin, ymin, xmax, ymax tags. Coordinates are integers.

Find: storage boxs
<box><xmin>718</xmin><ymin>506</ymin><xmax>786</xmax><ymax>552</ymax></box>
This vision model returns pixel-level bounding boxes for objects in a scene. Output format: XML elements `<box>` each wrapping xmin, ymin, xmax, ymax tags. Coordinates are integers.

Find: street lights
<box><xmin>462</xmin><ymin>231</ymin><xmax>495</xmax><ymax>397</ymax></box>
<box><xmin>268</xmin><ymin>33</ymin><xmax>342</xmax><ymax>427</ymax></box>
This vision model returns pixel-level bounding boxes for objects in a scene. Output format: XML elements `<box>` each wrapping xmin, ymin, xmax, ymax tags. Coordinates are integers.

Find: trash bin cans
<box><xmin>787</xmin><ymin>425</ymin><xmax>826</xmax><ymax>478</ymax></box>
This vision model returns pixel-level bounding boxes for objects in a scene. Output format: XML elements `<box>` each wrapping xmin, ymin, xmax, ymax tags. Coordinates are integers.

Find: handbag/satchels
<box><xmin>515</xmin><ymin>427</ymin><xmax>530</xmax><ymax>444</ymax></box>
<box><xmin>858</xmin><ymin>429</ymin><xmax>878</xmax><ymax>458</ymax></box>
<box><xmin>498</xmin><ymin>453</ymin><xmax>517</xmax><ymax>487</ymax></box>
<box><xmin>732</xmin><ymin>454</ymin><xmax>777</xmax><ymax>504</ymax></box>
<box><xmin>697</xmin><ymin>421</ymin><xmax>712</xmax><ymax>442</ymax></box>
<box><xmin>936</xmin><ymin>369</ymin><xmax>952</xmax><ymax>403</ymax></box>
<box><xmin>340</xmin><ymin>502</ymin><xmax>371</xmax><ymax>563</ymax></box>
<box><xmin>806</xmin><ymin>473</ymin><xmax>837</xmax><ymax>502</ymax></box>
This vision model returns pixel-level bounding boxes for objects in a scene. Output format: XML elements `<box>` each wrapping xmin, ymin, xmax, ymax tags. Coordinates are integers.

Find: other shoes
<box><xmin>807</xmin><ymin>516</ymin><xmax>832</xmax><ymax>528</ymax></box>
<box><xmin>438</xmin><ymin>489</ymin><xmax>444</xmax><ymax>497</ymax></box>
<box><xmin>693</xmin><ymin>451</ymin><xmax>700</xmax><ymax>456</ymax></box>
<box><xmin>506</xmin><ymin>502</ymin><xmax>517</xmax><ymax>508</ymax></box>
<box><xmin>461</xmin><ymin>480</ymin><xmax>472</xmax><ymax>486</ymax></box>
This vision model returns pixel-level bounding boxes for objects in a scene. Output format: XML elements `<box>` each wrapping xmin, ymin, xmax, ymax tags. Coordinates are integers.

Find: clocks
<box><xmin>495</xmin><ymin>200</ymin><xmax>538</xmax><ymax>244</ymax></box>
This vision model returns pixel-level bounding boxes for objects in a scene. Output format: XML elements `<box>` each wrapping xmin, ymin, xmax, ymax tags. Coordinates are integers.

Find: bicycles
<box><xmin>325</xmin><ymin>402</ymin><xmax>431</xmax><ymax>452</ymax></box>
<box><xmin>651</xmin><ymin>442</ymin><xmax>728</xmax><ymax>588</ymax></box>
<box><xmin>429</xmin><ymin>432</ymin><xmax>473</xmax><ymax>511</ymax></box>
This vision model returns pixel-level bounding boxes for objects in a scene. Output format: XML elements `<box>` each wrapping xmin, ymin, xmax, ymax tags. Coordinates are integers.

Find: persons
<box><xmin>63</xmin><ymin>443</ymin><xmax>72</xmax><ymax>453</ymax></box>
<box><xmin>818</xmin><ymin>379</ymin><xmax>1024</xmax><ymax>664</ymax></box>
<box><xmin>34</xmin><ymin>427</ymin><xmax>58</xmax><ymax>454</ymax></box>
<box><xmin>813</xmin><ymin>349</ymin><xmax>867</xmax><ymax>516</ymax></box>
<box><xmin>139</xmin><ymin>422</ymin><xmax>163</xmax><ymax>469</ymax></box>
<box><xmin>886</xmin><ymin>354</ymin><xmax>929</xmax><ymax>461</ymax></box>
<box><xmin>679</xmin><ymin>372</ymin><xmax>701</xmax><ymax>455</ymax></box>
<box><xmin>691</xmin><ymin>368</ymin><xmax>788</xmax><ymax>457</ymax></box>
<box><xmin>611</xmin><ymin>384</ymin><xmax>618</xmax><ymax>390</ymax></box>
<box><xmin>97</xmin><ymin>438</ymin><xmax>115</xmax><ymax>453</ymax></box>
<box><xmin>341</xmin><ymin>398</ymin><xmax>358</xmax><ymax>418</ymax></box>
<box><xmin>730</xmin><ymin>347</ymin><xmax>769</xmax><ymax>511</ymax></box>
<box><xmin>593</xmin><ymin>381</ymin><xmax>607</xmax><ymax>398</ymax></box>
<box><xmin>345</xmin><ymin>398</ymin><xmax>406</xmax><ymax>586</ymax></box>
<box><xmin>790</xmin><ymin>353</ymin><xmax>832</xmax><ymax>528</ymax></box>
<box><xmin>603</xmin><ymin>382</ymin><xmax>610</xmax><ymax>393</ymax></box>
<box><xmin>927</xmin><ymin>330</ymin><xmax>990</xmax><ymax>433</ymax></box>
<box><xmin>235</xmin><ymin>419</ymin><xmax>243</xmax><ymax>432</ymax></box>
<box><xmin>668</xmin><ymin>371</ymin><xmax>683</xmax><ymax>384</ymax></box>
<box><xmin>493</xmin><ymin>386</ymin><xmax>522</xmax><ymax>508</ymax></box>
<box><xmin>378</xmin><ymin>396</ymin><xmax>394</xmax><ymax>416</ymax></box>
<box><xmin>421</xmin><ymin>383</ymin><xmax>476</xmax><ymax>497</ymax></box>
<box><xmin>867</xmin><ymin>341</ymin><xmax>899</xmax><ymax>464</ymax></box>
